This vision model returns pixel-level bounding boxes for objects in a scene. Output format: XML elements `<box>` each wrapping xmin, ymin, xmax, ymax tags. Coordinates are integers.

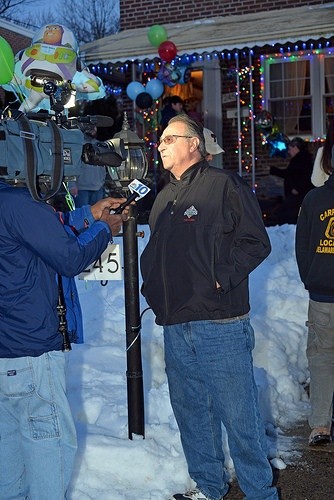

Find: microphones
<box><xmin>114</xmin><ymin>179</ymin><xmax>152</xmax><ymax>214</ymax></box>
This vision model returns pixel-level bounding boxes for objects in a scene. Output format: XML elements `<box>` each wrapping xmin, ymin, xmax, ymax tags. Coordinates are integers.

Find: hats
<box><xmin>203</xmin><ymin>127</ymin><xmax>225</xmax><ymax>155</ymax></box>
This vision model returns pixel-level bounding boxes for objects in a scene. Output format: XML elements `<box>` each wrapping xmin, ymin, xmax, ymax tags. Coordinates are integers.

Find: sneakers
<box><xmin>170</xmin><ymin>488</ymin><xmax>225</xmax><ymax>500</ymax></box>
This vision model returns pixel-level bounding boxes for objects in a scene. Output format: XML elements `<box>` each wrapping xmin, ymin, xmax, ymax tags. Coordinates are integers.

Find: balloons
<box><xmin>126</xmin><ymin>24</ymin><xmax>191</xmax><ymax>108</ymax></box>
<box><xmin>1</xmin><ymin>35</ymin><xmax>15</xmax><ymax>85</ymax></box>
<box><xmin>3</xmin><ymin>23</ymin><xmax>107</xmax><ymax>118</ymax></box>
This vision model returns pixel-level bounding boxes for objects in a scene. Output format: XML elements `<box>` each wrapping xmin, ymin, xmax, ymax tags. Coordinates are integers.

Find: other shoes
<box><xmin>310</xmin><ymin>429</ymin><xmax>333</xmax><ymax>445</ymax></box>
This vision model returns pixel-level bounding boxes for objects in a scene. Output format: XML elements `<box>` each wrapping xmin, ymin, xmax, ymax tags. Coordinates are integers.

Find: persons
<box><xmin>295</xmin><ymin>119</ymin><xmax>334</xmax><ymax>446</ymax></box>
<box><xmin>266</xmin><ymin>137</ymin><xmax>316</xmax><ymax>225</ymax></box>
<box><xmin>158</xmin><ymin>95</ymin><xmax>183</xmax><ymax>134</ymax></box>
<box><xmin>0</xmin><ymin>180</ymin><xmax>135</xmax><ymax>500</ymax></box>
<box><xmin>140</xmin><ymin>114</ymin><xmax>279</xmax><ymax>500</ymax></box>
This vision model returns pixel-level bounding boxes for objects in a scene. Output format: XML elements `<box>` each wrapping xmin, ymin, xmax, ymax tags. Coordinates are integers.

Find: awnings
<box><xmin>77</xmin><ymin>1</ymin><xmax>334</xmax><ymax>65</ymax></box>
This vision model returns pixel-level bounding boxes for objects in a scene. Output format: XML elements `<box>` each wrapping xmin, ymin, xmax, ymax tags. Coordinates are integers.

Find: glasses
<box><xmin>156</xmin><ymin>134</ymin><xmax>192</xmax><ymax>145</ymax></box>
